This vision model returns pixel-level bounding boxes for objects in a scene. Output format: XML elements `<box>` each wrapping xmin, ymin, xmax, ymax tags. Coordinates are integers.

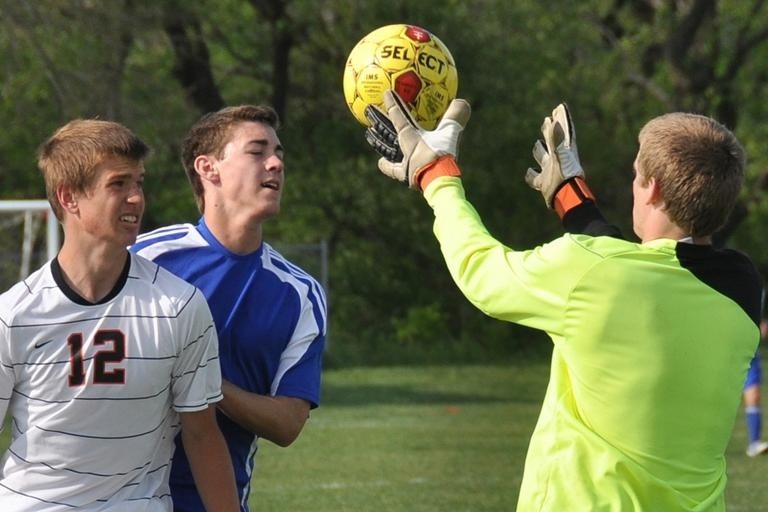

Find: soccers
<box><xmin>344</xmin><ymin>22</ymin><xmax>458</xmax><ymax>132</ymax></box>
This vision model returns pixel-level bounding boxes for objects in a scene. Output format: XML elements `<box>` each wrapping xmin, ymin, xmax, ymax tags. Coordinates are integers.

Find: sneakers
<box><xmin>746</xmin><ymin>441</ymin><xmax>767</xmax><ymax>458</ymax></box>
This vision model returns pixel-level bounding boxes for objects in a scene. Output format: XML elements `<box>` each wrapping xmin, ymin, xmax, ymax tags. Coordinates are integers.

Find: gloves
<box><xmin>524</xmin><ymin>101</ymin><xmax>595</xmax><ymax>221</ymax></box>
<box><xmin>364</xmin><ymin>89</ymin><xmax>471</xmax><ymax>194</ymax></box>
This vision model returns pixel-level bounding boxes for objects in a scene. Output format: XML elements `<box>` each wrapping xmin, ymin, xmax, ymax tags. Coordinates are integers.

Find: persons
<box><xmin>363</xmin><ymin>88</ymin><xmax>764</xmax><ymax>512</ymax></box>
<box><xmin>127</xmin><ymin>104</ymin><xmax>329</xmax><ymax>511</ymax></box>
<box><xmin>742</xmin><ymin>319</ymin><xmax>768</xmax><ymax>459</ymax></box>
<box><xmin>0</xmin><ymin>117</ymin><xmax>244</xmax><ymax>510</ymax></box>
